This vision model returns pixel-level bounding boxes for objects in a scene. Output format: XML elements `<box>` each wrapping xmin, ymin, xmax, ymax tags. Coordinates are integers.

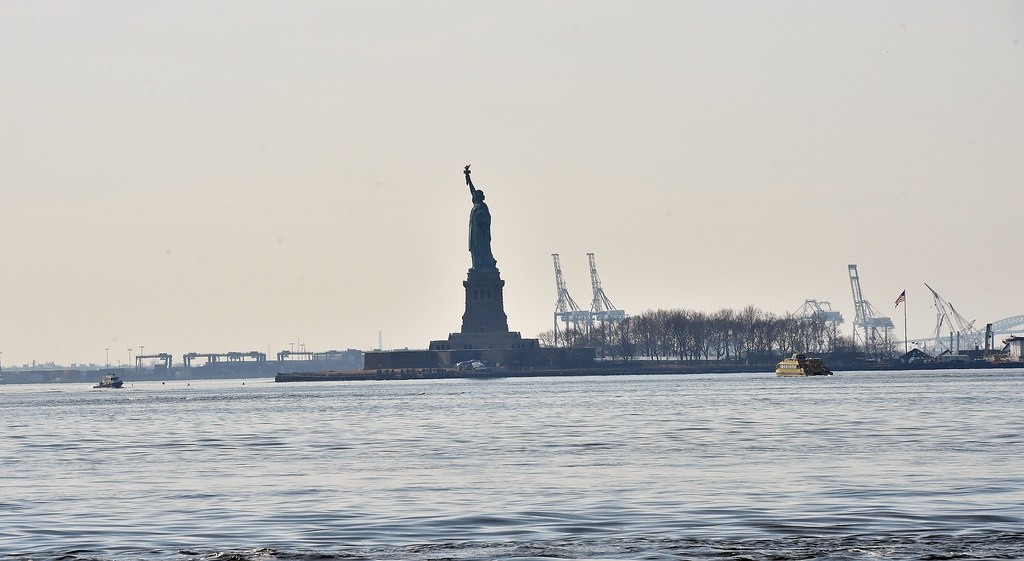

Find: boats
<box><xmin>93</xmin><ymin>373</ymin><xmax>123</xmax><ymax>389</ymax></box>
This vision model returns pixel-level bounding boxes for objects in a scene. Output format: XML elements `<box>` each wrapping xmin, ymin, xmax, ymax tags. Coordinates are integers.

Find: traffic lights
<box><xmin>776</xmin><ymin>353</ymin><xmax>833</xmax><ymax>377</ymax></box>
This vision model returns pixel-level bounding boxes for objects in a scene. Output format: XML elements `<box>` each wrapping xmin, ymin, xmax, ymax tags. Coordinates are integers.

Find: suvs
<box><xmin>458</xmin><ymin>359</ymin><xmax>486</xmax><ymax>371</ymax></box>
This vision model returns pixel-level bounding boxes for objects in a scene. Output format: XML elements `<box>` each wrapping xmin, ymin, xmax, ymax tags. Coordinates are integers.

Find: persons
<box><xmin>465</xmin><ymin>175</ymin><xmax>499</xmax><ymax>272</ymax></box>
